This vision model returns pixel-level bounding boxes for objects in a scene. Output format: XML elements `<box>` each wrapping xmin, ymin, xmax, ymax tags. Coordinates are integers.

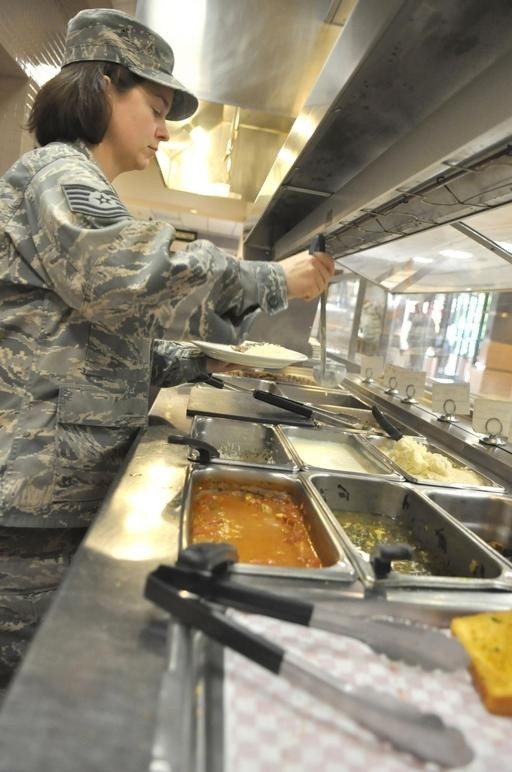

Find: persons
<box><xmin>0</xmin><ymin>7</ymin><xmax>335</xmax><ymax>696</ymax></box>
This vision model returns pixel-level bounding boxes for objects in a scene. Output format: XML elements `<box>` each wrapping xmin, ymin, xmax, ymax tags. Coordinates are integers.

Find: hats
<box><xmin>59</xmin><ymin>7</ymin><xmax>199</xmax><ymax>122</ymax></box>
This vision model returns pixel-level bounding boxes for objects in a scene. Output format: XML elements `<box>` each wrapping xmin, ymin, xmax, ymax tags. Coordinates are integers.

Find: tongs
<box><xmin>254</xmin><ymin>386</ymin><xmax>362</xmax><ymax>430</ymax></box>
<box><xmin>142</xmin><ymin>557</ymin><xmax>479</xmax><ymax>772</ymax></box>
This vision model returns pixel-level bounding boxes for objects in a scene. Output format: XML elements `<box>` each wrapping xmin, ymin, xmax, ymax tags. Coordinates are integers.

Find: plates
<box><xmin>187</xmin><ymin>338</ymin><xmax>309</xmax><ymax>371</ymax></box>
